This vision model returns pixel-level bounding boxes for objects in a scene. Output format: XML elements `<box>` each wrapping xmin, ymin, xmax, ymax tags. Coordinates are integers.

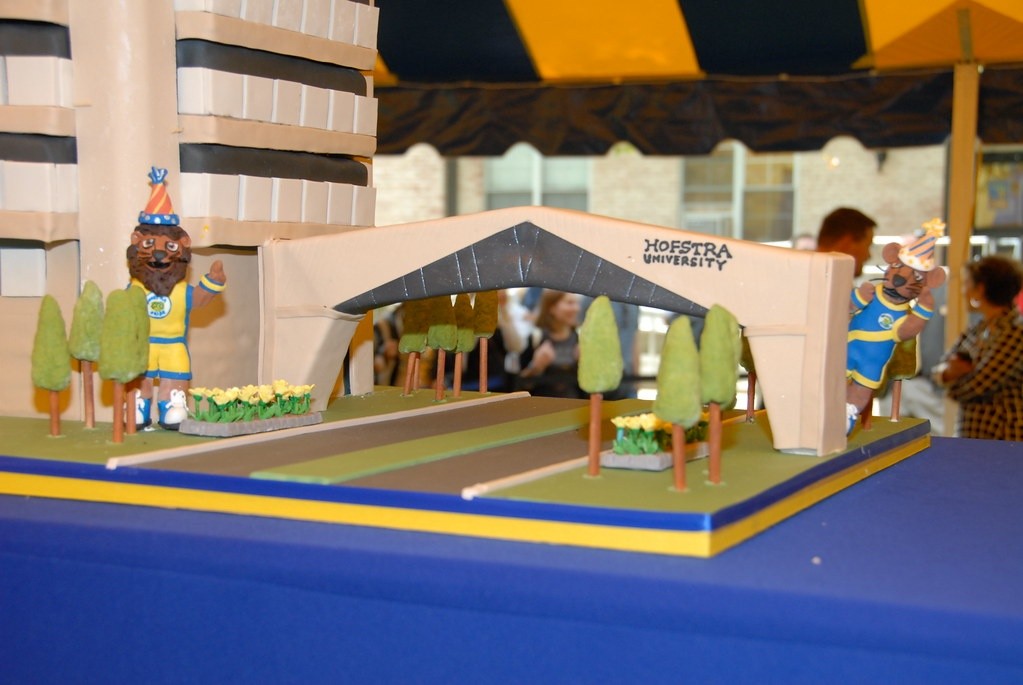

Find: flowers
<box><xmin>187</xmin><ymin>379</ymin><xmax>316</xmax><ymax>424</ymax></box>
<box><xmin>608</xmin><ymin>411</ymin><xmax>711</xmax><ymax>455</ymax></box>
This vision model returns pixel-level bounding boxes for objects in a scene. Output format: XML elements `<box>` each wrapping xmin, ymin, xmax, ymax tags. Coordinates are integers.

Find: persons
<box><xmin>373</xmin><ymin>287</ymin><xmax>628</xmax><ymax>400</ymax></box>
<box><xmin>928</xmin><ymin>253</ymin><xmax>1023</xmax><ymax>442</ymax></box>
<box><xmin>814</xmin><ymin>207</ymin><xmax>879</xmax><ymax>278</ymax></box>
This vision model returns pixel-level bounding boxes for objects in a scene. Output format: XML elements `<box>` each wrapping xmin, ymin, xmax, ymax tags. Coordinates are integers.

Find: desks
<box><xmin>0</xmin><ymin>433</ymin><xmax>1023</xmax><ymax>685</ymax></box>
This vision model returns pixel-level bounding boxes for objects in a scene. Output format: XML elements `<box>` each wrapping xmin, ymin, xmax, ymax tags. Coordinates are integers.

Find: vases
<box><xmin>178</xmin><ymin>411</ymin><xmax>323</xmax><ymax>439</ymax></box>
<box><xmin>598</xmin><ymin>442</ymin><xmax>708</xmax><ymax>473</ymax></box>
